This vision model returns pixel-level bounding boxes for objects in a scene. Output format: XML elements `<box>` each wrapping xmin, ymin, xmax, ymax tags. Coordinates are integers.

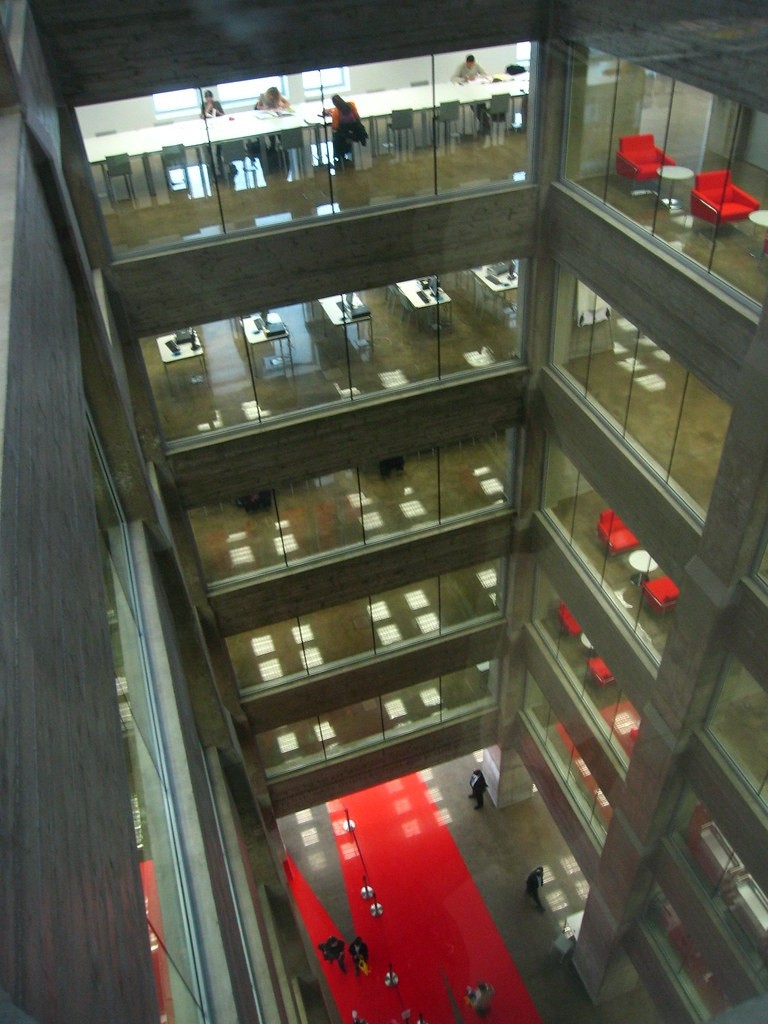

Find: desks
<box><xmin>156</xmin><ymin>329</ymin><xmax>209</xmax><ymax>396</ymax></box>
<box><xmin>317</xmin><ymin>292</ymin><xmax>374</xmax><ymax>359</ymax></box>
<box><xmin>656</xmin><ymin>165</ymin><xmax>695</xmax><ymax>210</ymax></box>
<box><xmin>748</xmin><ymin>210</ymin><xmax>768</xmax><ymax>260</ymax></box>
<box><xmin>239</xmin><ymin>313</ymin><xmax>293</xmax><ymax>378</ymax></box>
<box><xmin>629</xmin><ymin>548</ymin><xmax>658</xmax><ymax>586</ymax></box>
<box><xmin>580</xmin><ymin>632</ymin><xmax>596</xmax><ymax>657</ymax></box>
<box><xmin>82</xmin><ymin>60</ymin><xmax>656</xmax><ymax>196</ymax></box>
<box><xmin>469</xmin><ymin>264</ymin><xmax>519</xmax><ymax>326</ymax></box>
<box><xmin>396</xmin><ymin>279</ymin><xmax>452</xmax><ymax>333</ymax></box>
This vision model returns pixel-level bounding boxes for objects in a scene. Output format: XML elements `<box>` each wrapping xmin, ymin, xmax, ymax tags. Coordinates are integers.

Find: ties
<box><xmin>472</xmin><ymin>777</ymin><xmax>476</xmax><ymax>782</ymax></box>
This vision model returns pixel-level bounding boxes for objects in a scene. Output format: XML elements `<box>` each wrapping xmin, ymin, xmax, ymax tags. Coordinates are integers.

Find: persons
<box><xmin>318</xmin><ymin>937</ymin><xmax>369</xmax><ymax>976</ymax></box>
<box><xmin>467</xmin><ymin>768</ymin><xmax>489</xmax><ymax>811</ymax></box>
<box><xmin>453</xmin><ymin>54</ymin><xmax>492</xmax><ymax>129</ymax></box>
<box><xmin>331</xmin><ymin>93</ymin><xmax>368</xmax><ymax>170</ymax></box>
<box><xmin>465</xmin><ymin>980</ymin><xmax>495</xmax><ymax>1018</ymax></box>
<box><xmin>256</xmin><ymin>87</ymin><xmax>291</xmax><ymax>149</ymax></box>
<box><xmin>355</xmin><ymin>422</ymin><xmax>406</xmax><ymax>481</ymax></box>
<box><xmin>200</xmin><ymin>90</ymin><xmax>228</xmax><ymax>158</ymax></box>
<box><xmin>229</xmin><ymin>450</ymin><xmax>274</xmax><ymax>515</ymax></box>
<box><xmin>526</xmin><ymin>867</ymin><xmax>547</xmax><ymax>914</ymax></box>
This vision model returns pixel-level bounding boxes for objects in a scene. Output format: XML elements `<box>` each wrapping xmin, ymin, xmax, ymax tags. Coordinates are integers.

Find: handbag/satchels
<box><xmin>359</xmin><ymin>959</ymin><xmax>368</xmax><ymax>975</ymax></box>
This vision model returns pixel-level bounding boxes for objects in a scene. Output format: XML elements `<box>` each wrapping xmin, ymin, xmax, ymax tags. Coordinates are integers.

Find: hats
<box><xmin>473</xmin><ymin>768</ymin><xmax>480</xmax><ymax>773</ymax></box>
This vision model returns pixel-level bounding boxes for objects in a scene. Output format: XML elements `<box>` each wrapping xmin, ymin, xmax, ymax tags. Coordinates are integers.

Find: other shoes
<box><xmin>469</xmin><ymin>795</ymin><xmax>475</xmax><ymax>798</ymax></box>
<box><xmin>475</xmin><ymin>806</ymin><xmax>481</xmax><ymax>809</ymax></box>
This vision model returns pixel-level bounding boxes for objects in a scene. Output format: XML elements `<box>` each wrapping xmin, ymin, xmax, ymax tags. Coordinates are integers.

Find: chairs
<box><xmin>761</xmin><ymin>232</ymin><xmax>768</xmax><ymax>258</ymax></box>
<box><xmin>639</xmin><ymin>576</ymin><xmax>681</xmax><ymax>614</ymax></box>
<box><xmin>616</xmin><ymin>134</ymin><xmax>676</xmax><ymax>197</ymax></box>
<box><xmin>596</xmin><ymin>509</ymin><xmax>640</xmax><ymax>555</ymax></box>
<box><xmin>385</xmin><ymin>275</ymin><xmax>499</xmax><ymax>322</ymax></box>
<box><xmin>95</xmin><ymin>80</ymin><xmax>528</xmax><ymax>205</ymax></box>
<box><xmin>587</xmin><ymin>657</ymin><xmax>616</xmax><ymax>687</ymax></box>
<box><xmin>558</xmin><ymin>599</ymin><xmax>582</xmax><ymax>637</ymax></box>
<box><xmin>691</xmin><ymin>170</ymin><xmax>760</xmax><ymax>247</ymax></box>
